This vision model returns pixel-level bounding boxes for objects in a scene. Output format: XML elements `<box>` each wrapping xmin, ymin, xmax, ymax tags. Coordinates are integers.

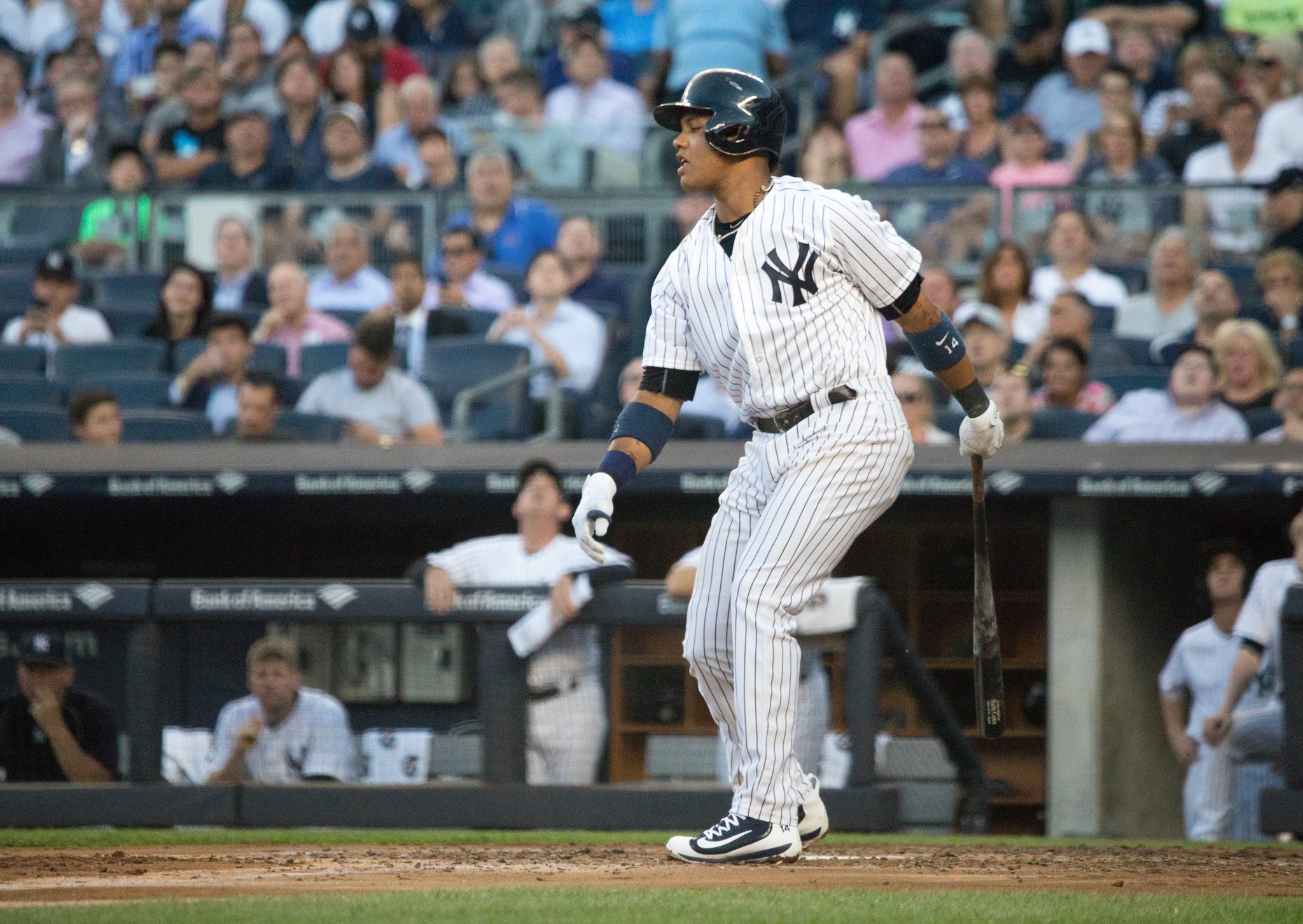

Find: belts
<box><xmin>529</xmin><ymin>684</ymin><xmax>577</xmax><ymax>698</ymax></box>
<box><xmin>758</xmin><ymin>387</ymin><xmax>854</xmax><ymax>435</ymax></box>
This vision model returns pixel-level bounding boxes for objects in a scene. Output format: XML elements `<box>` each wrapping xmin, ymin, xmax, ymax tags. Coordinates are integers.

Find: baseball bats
<box><xmin>971</xmin><ymin>453</ymin><xmax>1009</xmax><ymax>739</ymax></box>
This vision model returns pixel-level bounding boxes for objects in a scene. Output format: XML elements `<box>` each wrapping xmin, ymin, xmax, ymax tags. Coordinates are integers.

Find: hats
<box><xmin>21</xmin><ymin>638</ymin><xmax>76</xmax><ymax>669</ymax></box>
<box><xmin>952</xmin><ymin>304</ymin><xmax>1011</xmax><ymax>331</ymax></box>
<box><xmin>1066</xmin><ymin>22</ymin><xmax>1110</xmax><ymax>57</ymax></box>
<box><xmin>348</xmin><ymin>5</ymin><xmax>383</xmax><ymax>37</ymax></box>
<box><xmin>39</xmin><ymin>249</ymin><xmax>79</xmax><ymax>280</ymax></box>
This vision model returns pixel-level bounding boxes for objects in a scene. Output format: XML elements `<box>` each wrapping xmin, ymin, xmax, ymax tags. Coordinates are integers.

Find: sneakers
<box><xmin>663</xmin><ymin>809</ymin><xmax>802</xmax><ymax>864</ymax></box>
<box><xmin>796</xmin><ymin>797</ymin><xmax>829</xmax><ymax>848</ymax></box>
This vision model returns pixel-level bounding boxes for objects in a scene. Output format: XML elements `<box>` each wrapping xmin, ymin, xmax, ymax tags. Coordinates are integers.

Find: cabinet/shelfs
<box><xmin>607</xmin><ymin>516</ymin><xmax>1046</xmax><ymax>837</ymax></box>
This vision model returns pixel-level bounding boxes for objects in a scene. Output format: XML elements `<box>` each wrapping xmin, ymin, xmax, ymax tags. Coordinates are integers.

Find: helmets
<box><xmin>654</xmin><ymin>68</ymin><xmax>785</xmax><ymax>171</ymax></box>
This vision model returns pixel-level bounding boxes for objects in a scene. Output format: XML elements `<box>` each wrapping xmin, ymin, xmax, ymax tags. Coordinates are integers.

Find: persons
<box><xmin>573</xmin><ymin>68</ymin><xmax>1006</xmax><ymax>862</ymax></box>
<box><xmin>2</xmin><ymin>1</ymin><xmax>1303</xmax><ymax>868</ymax></box>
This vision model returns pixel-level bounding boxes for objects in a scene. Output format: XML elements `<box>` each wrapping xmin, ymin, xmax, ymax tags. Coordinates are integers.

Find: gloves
<box><xmin>568</xmin><ymin>471</ymin><xmax>617</xmax><ymax>565</ymax></box>
<box><xmin>957</xmin><ymin>399</ymin><xmax>1004</xmax><ymax>459</ymax></box>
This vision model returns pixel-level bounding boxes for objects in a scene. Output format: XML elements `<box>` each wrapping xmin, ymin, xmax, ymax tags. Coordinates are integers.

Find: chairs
<box><xmin>0</xmin><ymin>9</ymin><xmax>1303</xmax><ymax>453</ymax></box>
